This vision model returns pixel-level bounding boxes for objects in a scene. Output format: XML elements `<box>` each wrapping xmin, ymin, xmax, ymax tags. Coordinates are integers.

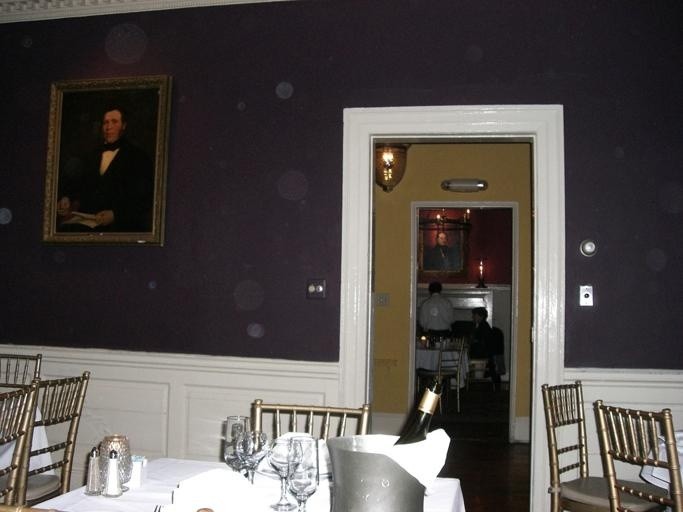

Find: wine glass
<box><xmin>224</xmin><ymin>414</ymin><xmax>319</xmax><ymax>512</ymax></box>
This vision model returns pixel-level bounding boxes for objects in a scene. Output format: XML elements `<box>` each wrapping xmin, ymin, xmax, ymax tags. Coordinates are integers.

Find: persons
<box><xmin>56</xmin><ymin>106</ymin><xmax>146</xmax><ymax>232</ymax></box>
<box><xmin>468</xmin><ymin>307</ymin><xmax>498</xmax><ymax>344</ymax></box>
<box><xmin>418</xmin><ymin>282</ymin><xmax>456</xmax><ymax>336</ymax></box>
<box><xmin>430</xmin><ymin>232</ymin><xmax>453</xmax><ymax>268</ymax></box>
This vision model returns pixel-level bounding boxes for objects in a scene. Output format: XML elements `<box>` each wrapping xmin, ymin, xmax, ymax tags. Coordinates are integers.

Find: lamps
<box><xmin>437</xmin><ymin>208</ymin><xmax>448</xmax><ymax>222</ymax></box>
<box><xmin>462</xmin><ymin>207</ymin><xmax>471</xmax><ymax>224</ymax></box>
<box><xmin>373</xmin><ymin>142</ymin><xmax>411</xmax><ymax>194</ymax></box>
<box><xmin>475</xmin><ymin>256</ymin><xmax>489</xmax><ymax>288</ymax></box>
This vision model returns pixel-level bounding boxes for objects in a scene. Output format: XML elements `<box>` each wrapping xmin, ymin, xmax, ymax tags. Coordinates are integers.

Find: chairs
<box><xmin>1</xmin><ymin>352</ymin><xmax>90</xmax><ymax>511</ymax></box>
<box><xmin>248</xmin><ymin>397</ymin><xmax>373</xmax><ymax>447</ymax></box>
<box><xmin>543</xmin><ymin>379</ymin><xmax>670</xmax><ymax>512</ymax></box>
<box><xmin>592</xmin><ymin>396</ymin><xmax>683</xmax><ymax>512</ymax></box>
<box><xmin>417</xmin><ymin>333</ymin><xmax>466</xmax><ymax>415</ymax></box>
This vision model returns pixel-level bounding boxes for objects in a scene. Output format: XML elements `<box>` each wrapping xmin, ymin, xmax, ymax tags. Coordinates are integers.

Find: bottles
<box><xmin>392</xmin><ymin>378</ymin><xmax>443</xmax><ymax>446</ymax></box>
<box><xmin>87</xmin><ymin>435</ymin><xmax>133</xmax><ymax>497</ymax></box>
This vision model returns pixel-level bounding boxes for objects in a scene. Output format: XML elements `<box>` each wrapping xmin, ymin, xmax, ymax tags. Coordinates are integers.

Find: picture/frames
<box><xmin>39</xmin><ymin>73</ymin><xmax>173</xmax><ymax>248</ymax></box>
<box><xmin>419</xmin><ymin>218</ymin><xmax>467</xmax><ymax>279</ymax></box>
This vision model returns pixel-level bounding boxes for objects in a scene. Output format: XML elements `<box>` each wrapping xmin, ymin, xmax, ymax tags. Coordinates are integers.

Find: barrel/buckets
<box><xmin>325</xmin><ymin>434</ymin><xmax>425</xmax><ymax>512</ymax></box>
